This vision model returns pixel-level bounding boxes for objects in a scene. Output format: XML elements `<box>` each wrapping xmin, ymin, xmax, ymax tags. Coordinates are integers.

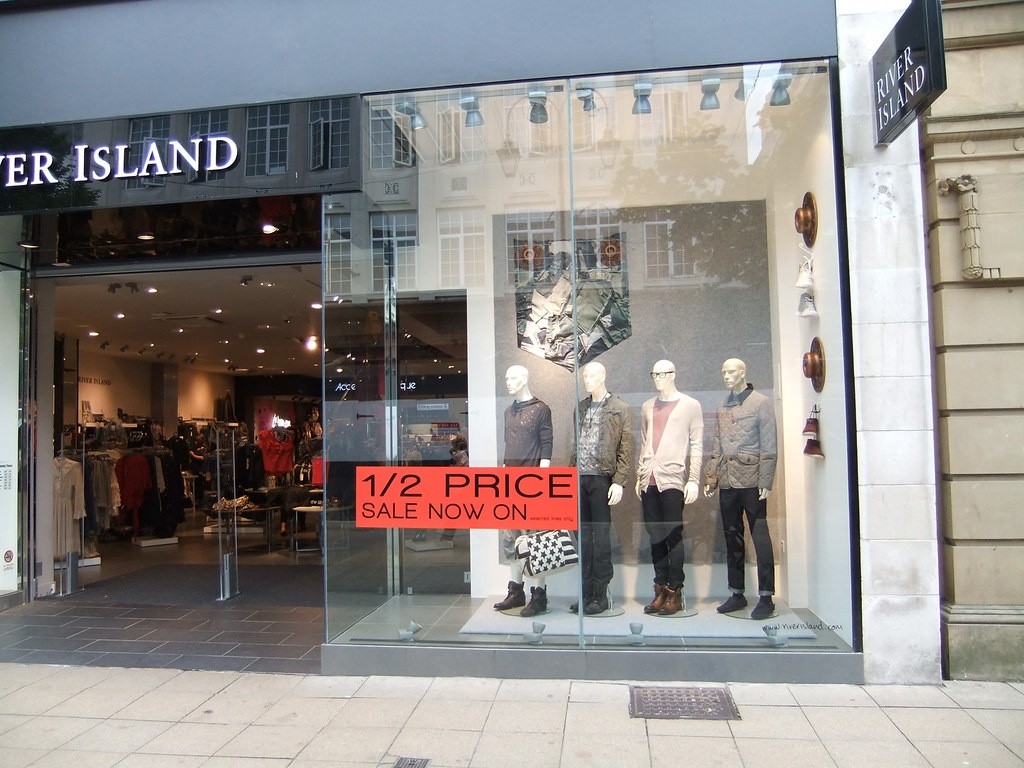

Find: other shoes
<box><xmin>570</xmin><ymin>596</ymin><xmax>591</xmax><ymax>611</ymax></box>
<box><xmin>803</xmin><ymin>439</ymin><xmax>825</xmax><ymax>459</ymax></box>
<box><xmin>803</xmin><ymin>418</ymin><xmax>818</xmax><ymax>436</ymax></box>
<box><xmin>585</xmin><ymin>591</ymin><xmax>608</xmax><ymax>614</ymax></box>
<box><xmin>751</xmin><ymin>597</ymin><xmax>775</xmax><ymax>619</ymax></box>
<box><xmin>717</xmin><ymin>595</ymin><xmax>747</xmax><ymax>613</ymax></box>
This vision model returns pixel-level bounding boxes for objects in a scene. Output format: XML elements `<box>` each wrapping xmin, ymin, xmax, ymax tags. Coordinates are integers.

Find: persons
<box><xmin>702</xmin><ymin>358</ymin><xmax>779</xmax><ymax>619</ymax></box>
<box><xmin>493</xmin><ymin>365</ymin><xmax>553</xmax><ymax>617</ymax></box>
<box><xmin>568</xmin><ymin>361</ymin><xmax>634</xmax><ymax>615</ymax></box>
<box><xmin>635</xmin><ymin>359</ymin><xmax>704</xmax><ymax>616</ymax></box>
<box><xmin>188</xmin><ymin>432</ymin><xmax>211</xmax><ymax>509</ymax></box>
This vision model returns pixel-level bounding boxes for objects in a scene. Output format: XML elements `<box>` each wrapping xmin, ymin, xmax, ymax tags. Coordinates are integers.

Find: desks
<box><xmin>293</xmin><ymin>505</ymin><xmax>355</xmax><ymax>552</ymax></box>
<box><xmin>215</xmin><ymin>505</ymin><xmax>281</xmax><ymax>557</ymax></box>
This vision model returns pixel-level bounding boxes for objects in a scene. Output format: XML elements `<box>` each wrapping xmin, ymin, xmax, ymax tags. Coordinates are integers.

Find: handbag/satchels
<box><xmin>514</xmin><ymin>530</ymin><xmax>578</xmax><ymax>578</ymax></box>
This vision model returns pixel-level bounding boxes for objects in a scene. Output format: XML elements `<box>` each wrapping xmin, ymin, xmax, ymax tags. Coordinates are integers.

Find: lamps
<box><xmin>761</xmin><ymin>623</ymin><xmax>789</xmax><ymax>648</ymax></box>
<box><xmin>107</xmin><ymin>282</ymin><xmax>122</xmax><ymax>294</ymax></box>
<box><xmin>529</xmin><ymin>103</ymin><xmax>548</xmax><ymax>124</ymax></box>
<box><xmin>125</xmin><ymin>282</ymin><xmax>140</xmax><ymax>294</ymax></box>
<box><xmin>583</xmin><ymin>98</ymin><xmax>597</xmax><ymax>111</ymax></box>
<box><xmin>523</xmin><ymin>622</ymin><xmax>547</xmax><ymax>646</ymax></box>
<box><xmin>396</xmin><ymin>620</ymin><xmax>423</xmax><ymax>644</ymax></box>
<box><xmin>700</xmin><ymin>89</ymin><xmax>720</xmax><ymax>110</ymax></box>
<box><xmin>239</xmin><ymin>276</ymin><xmax>253</xmax><ymax>287</ymax></box>
<box><xmin>631</xmin><ymin>96</ymin><xmax>652</xmax><ymax>114</ymax></box>
<box><xmin>734</xmin><ymin>80</ymin><xmax>744</xmax><ymax>101</ymax></box>
<box><xmin>770</xmin><ymin>86</ymin><xmax>790</xmax><ymax>106</ymax></box>
<box><xmin>119</xmin><ymin>345</ymin><xmax>129</xmax><ymax>352</ymax></box>
<box><xmin>411</xmin><ymin>113</ymin><xmax>428</xmax><ymax>131</ymax></box>
<box><xmin>466</xmin><ymin>110</ymin><xmax>484</xmax><ymax>127</ymax></box>
<box><xmin>136</xmin><ymin>347</ymin><xmax>146</xmax><ymax>356</ymax></box>
<box><xmin>626</xmin><ymin>623</ymin><xmax>646</xmax><ymax>646</ymax></box>
<box><xmin>99</xmin><ymin>342</ymin><xmax>109</xmax><ymax>350</ymax></box>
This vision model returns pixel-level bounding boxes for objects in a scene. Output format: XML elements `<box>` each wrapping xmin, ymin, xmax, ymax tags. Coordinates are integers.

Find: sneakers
<box><xmin>798</xmin><ymin>292</ymin><xmax>817</xmax><ymax>317</ymax></box>
<box><xmin>796</xmin><ymin>242</ymin><xmax>813</xmax><ymax>287</ymax></box>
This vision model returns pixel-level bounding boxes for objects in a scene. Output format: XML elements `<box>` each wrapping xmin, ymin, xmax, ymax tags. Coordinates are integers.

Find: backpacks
<box><xmin>293</xmin><ymin>454</ymin><xmax>312</xmax><ymax>484</ymax></box>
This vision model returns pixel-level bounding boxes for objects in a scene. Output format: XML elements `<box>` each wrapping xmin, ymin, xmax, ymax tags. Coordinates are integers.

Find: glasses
<box><xmin>650</xmin><ymin>371</ymin><xmax>675</xmax><ymax>379</ymax></box>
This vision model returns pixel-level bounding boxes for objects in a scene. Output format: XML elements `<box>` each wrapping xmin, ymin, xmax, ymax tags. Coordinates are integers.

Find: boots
<box><xmin>659</xmin><ymin>586</ymin><xmax>682</xmax><ymax>615</ymax></box>
<box><xmin>644</xmin><ymin>584</ymin><xmax>666</xmax><ymax>613</ymax></box>
<box><xmin>493</xmin><ymin>581</ymin><xmax>526</xmax><ymax>609</ymax></box>
<box><xmin>520</xmin><ymin>585</ymin><xmax>547</xmax><ymax>615</ymax></box>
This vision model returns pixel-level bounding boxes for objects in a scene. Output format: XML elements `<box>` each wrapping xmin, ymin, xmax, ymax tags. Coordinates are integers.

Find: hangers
<box><xmin>54</xmin><ymin>444</ymin><xmax>173</xmax><ymax>461</ymax></box>
